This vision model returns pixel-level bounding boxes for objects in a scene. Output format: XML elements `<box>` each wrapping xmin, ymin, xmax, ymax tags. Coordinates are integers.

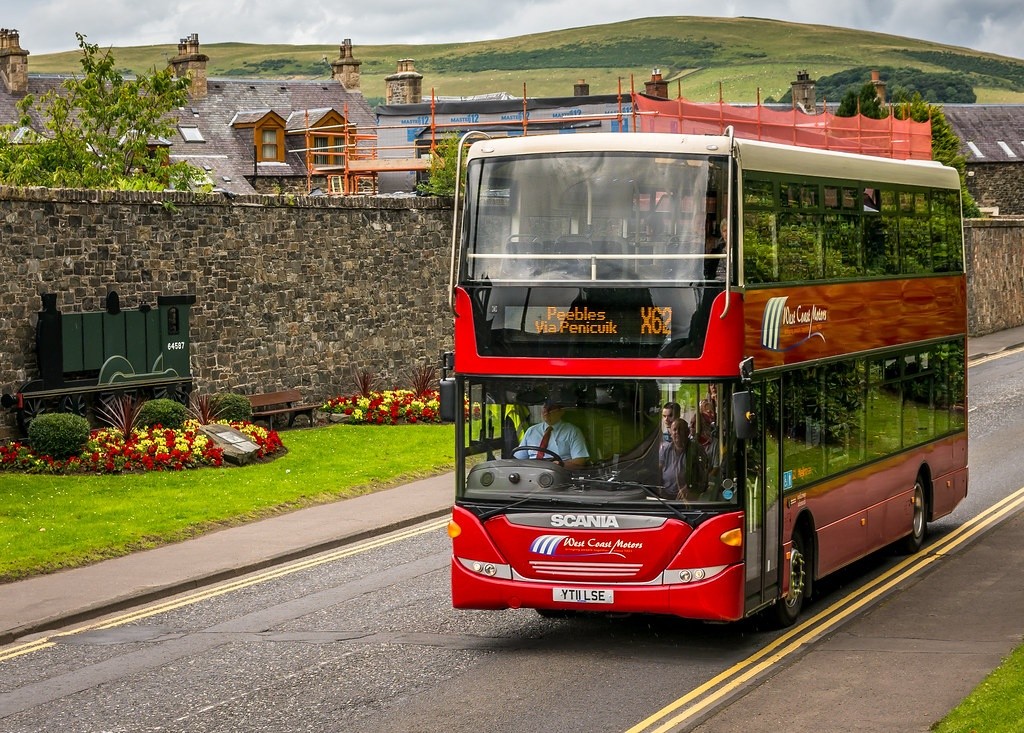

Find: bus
<box><xmin>438</xmin><ymin>129</ymin><xmax>969</xmax><ymax>632</ymax></box>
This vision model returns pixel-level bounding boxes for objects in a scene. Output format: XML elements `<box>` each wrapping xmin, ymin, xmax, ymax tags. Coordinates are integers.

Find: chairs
<box><xmin>553</xmin><ymin>236</ymin><xmax>595</xmax><ymax>281</ymax></box>
<box><xmin>661</xmin><ymin>234</ymin><xmax>703</xmax><ymax>281</ymax></box>
<box><xmin>594</xmin><ymin>234</ymin><xmax>628</xmax><ymax>279</ymax></box>
<box><xmin>501</xmin><ymin>234</ymin><xmax>546</xmax><ymax>275</ymax></box>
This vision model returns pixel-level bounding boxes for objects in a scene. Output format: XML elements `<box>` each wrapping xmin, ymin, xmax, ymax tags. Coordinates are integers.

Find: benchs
<box><xmin>244</xmin><ymin>390</ymin><xmax>324</xmax><ymax>431</ymax></box>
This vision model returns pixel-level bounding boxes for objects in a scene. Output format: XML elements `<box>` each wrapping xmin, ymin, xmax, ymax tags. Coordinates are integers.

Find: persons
<box><xmin>691</xmin><ymin>213</ymin><xmax>719</xmax><ymax>254</ymax></box>
<box><xmin>715</xmin><ymin>219</ymin><xmax>733</xmax><ymax>281</ymax></box>
<box><xmin>630</xmin><ymin>215</ymin><xmax>666</xmax><ymax>272</ymax></box>
<box><xmin>512</xmin><ymin>392</ymin><xmax>591</xmax><ymax>480</ymax></box>
<box><xmin>690</xmin><ymin>383</ymin><xmax>730</xmax><ymax>481</ymax></box>
<box><xmin>659</xmin><ymin>419</ymin><xmax>707</xmax><ymax>503</ymax></box>
<box><xmin>658</xmin><ymin>402</ymin><xmax>682</xmax><ymax>458</ymax></box>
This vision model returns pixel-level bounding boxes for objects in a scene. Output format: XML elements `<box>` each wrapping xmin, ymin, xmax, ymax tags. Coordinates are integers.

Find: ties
<box><xmin>536</xmin><ymin>426</ymin><xmax>553</xmax><ymax>461</ymax></box>
